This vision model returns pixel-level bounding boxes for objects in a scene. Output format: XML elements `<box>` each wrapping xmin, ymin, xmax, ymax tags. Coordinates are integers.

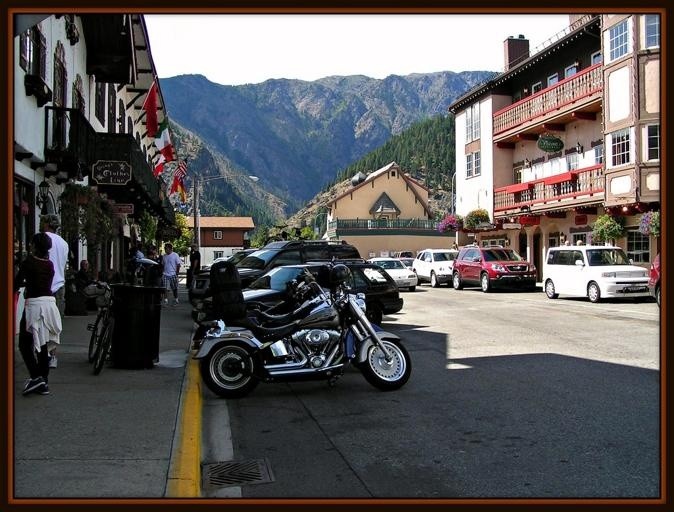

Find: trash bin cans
<box><xmin>108</xmin><ymin>283</ymin><xmax>166</xmax><ymax>370</ymax></box>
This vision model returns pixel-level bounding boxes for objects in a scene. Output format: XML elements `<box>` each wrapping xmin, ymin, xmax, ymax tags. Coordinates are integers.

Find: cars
<box><xmin>225</xmin><ymin>248</ymin><xmax>260</xmax><ymax>265</ymax></box>
<box><xmin>192</xmin><ymin>259</ymin><xmax>405</xmax><ymax>334</ymax></box>
<box><xmin>363</xmin><ymin>258</ymin><xmax>417</xmax><ymax>291</ymax></box>
<box><xmin>393</xmin><ymin>251</ymin><xmax>414</xmax><ymax>259</ymax></box>
<box><xmin>647</xmin><ymin>253</ymin><xmax>660</xmax><ymax>306</ymax></box>
<box><xmin>203</xmin><ymin>257</ymin><xmax>229</xmax><ymax>270</ymax></box>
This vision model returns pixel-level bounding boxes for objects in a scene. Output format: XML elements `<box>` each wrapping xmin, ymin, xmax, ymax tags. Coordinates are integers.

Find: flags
<box><xmin>142</xmin><ymin>81</ymin><xmax>188</xmax><ymax>203</ymax></box>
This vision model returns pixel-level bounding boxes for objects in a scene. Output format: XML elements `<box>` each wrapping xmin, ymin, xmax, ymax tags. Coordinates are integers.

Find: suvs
<box><xmin>188</xmin><ymin>236</ymin><xmax>361</xmax><ymax>308</ymax></box>
<box><xmin>451</xmin><ymin>244</ymin><xmax>537</xmax><ymax>292</ymax></box>
<box><xmin>411</xmin><ymin>249</ymin><xmax>458</xmax><ymax>287</ymax></box>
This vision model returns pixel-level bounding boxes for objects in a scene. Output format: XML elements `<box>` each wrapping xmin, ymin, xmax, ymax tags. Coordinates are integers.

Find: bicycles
<box><xmin>85</xmin><ymin>279</ymin><xmax>123</xmax><ymax>375</ymax></box>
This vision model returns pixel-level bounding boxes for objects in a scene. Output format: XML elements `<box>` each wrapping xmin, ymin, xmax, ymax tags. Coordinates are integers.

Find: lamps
<box><xmin>575</xmin><ymin>141</ymin><xmax>584</xmax><ymax>158</ymax></box>
<box><xmin>524</xmin><ymin>158</ymin><xmax>532</xmax><ymax>173</ymax></box>
<box><xmin>35</xmin><ymin>172</ymin><xmax>50</xmax><ymax>217</ymax></box>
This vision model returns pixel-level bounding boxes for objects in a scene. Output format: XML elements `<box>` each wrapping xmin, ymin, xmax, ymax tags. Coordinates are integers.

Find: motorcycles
<box><xmin>192</xmin><ymin>256</ymin><xmax>412</xmax><ymax>391</ymax></box>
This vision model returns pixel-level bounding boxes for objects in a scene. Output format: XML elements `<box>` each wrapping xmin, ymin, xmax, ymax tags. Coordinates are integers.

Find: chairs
<box><xmin>65</xmin><ymin>278</ymin><xmax>99</xmax><ymax>315</ymax></box>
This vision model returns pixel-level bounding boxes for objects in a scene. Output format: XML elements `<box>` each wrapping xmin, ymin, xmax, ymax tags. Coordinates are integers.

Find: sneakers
<box><xmin>22</xmin><ymin>376</ymin><xmax>50</xmax><ymax>395</ymax></box>
<box><xmin>49</xmin><ymin>356</ymin><xmax>57</xmax><ymax>368</ymax></box>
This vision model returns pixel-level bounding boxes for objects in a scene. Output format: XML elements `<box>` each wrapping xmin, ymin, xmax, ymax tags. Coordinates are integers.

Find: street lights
<box><xmin>192</xmin><ymin>174</ymin><xmax>259</xmax><ymax>251</ymax></box>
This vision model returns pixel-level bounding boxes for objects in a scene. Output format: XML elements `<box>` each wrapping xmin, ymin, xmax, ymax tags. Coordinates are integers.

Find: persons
<box><xmin>38</xmin><ymin>212</ymin><xmax>71</xmax><ymax>367</ymax></box>
<box><xmin>77</xmin><ymin>243</ymin><xmax>200</xmax><ymax>308</ymax></box>
<box><xmin>17</xmin><ymin>232</ymin><xmax>64</xmax><ymax>397</ymax></box>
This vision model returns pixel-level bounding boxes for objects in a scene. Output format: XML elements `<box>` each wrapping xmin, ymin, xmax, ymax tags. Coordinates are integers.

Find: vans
<box><xmin>541</xmin><ymin>245</ymin><xmax>649</xmax><ymax>303</ymax></box>
